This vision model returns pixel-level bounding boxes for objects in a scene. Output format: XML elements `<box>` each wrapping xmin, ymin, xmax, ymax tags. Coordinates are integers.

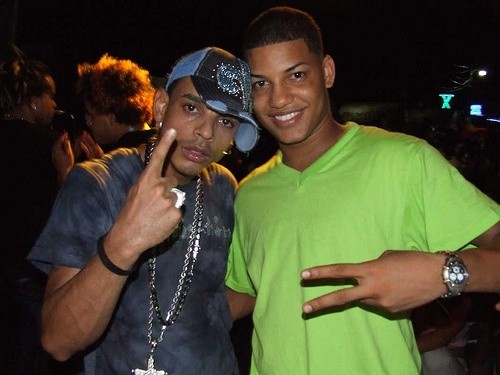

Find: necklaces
<box><xmin>134</xmin><ymin>134</ymin><xmax>205</xmax><ymax>375</ymax></box>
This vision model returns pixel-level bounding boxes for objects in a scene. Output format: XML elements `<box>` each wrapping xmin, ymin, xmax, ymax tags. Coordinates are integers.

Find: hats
<box><xmin>167</xmin><ymin>45</ymin><xmax>258</xmax><ymax>152</ymax></box>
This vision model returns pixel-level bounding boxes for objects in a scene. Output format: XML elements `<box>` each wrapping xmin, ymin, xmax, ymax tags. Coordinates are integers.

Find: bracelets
<box><xmin>97</xmin><ymin>232</ymin><xmax>133</xmax><ymax>276</ymax></box>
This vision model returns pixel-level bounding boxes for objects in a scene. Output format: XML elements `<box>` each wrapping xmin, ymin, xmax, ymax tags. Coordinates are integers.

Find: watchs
<box><xmin>436</xmin><ymin>250</ymin><xmax>469</xmax><ymax>297</ymax></box>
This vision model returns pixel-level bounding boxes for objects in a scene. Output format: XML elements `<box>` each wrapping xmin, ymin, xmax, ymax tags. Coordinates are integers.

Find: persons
<box><xmin>24</xmin><ymin>46</ymin><xmax>261</xmax><ymax>375</ymax></box>
<box><xmin>0</xmin><ymin>42</ymin><xmax>158</xmax><ymax>375</ymax></box>
<box><xmin>410</xmin><ymin>120</ymin><xmax>500</xmax><ymax>375</ymax></box>
<box><xmin>224</xmin><ymin>6</ymin><xmax>500</xmax><ymax>375</ymax></box>
